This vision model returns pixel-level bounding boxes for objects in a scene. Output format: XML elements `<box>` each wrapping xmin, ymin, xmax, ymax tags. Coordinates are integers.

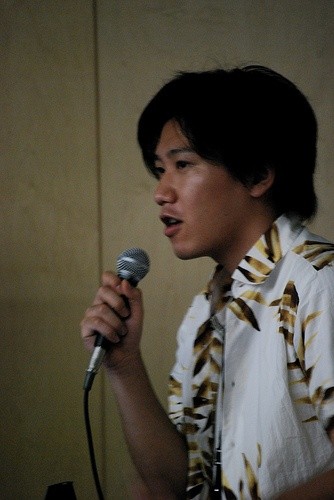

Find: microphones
<box><xmin>85</xmin><ymin>247</ymin><xmax>151</xmax><ymax>391</ymax></box>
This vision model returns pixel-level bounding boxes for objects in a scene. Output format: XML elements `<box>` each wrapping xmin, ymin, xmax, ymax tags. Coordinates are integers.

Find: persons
<box><xmin>80</xmin><ymin>66</ymin><xmax>334</xmax><ymax>500</ymax></box>
